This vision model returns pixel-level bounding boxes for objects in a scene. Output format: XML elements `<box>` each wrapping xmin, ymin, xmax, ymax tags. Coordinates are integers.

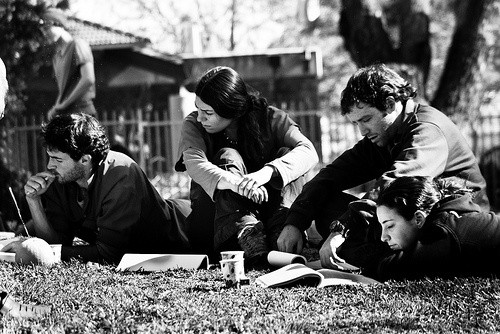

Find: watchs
<box><xmin>330</xmin><ymin>220</ymin><xmax>350</xmax><ymax>239</ymax></box>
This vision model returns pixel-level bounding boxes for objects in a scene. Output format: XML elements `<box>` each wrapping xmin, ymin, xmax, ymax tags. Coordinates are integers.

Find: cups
<box><xmin>219</xmin><ymin>250</ymin><xmax>245</xmax><ymax>285</ymax></box>
<box><xmin>48</xmin><ymin>243</ymin><xmax>62</xmax><ymax>264</ymax></box>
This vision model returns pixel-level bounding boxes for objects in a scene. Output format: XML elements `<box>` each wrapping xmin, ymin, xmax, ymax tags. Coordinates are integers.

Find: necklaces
<box><xmin>224</xmin><ymin>133</ymin><xmax>238</xmax><ymax>145</ymax></box>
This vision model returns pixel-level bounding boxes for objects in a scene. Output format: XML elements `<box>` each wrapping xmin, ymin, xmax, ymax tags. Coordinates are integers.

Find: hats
<box><xmin>39</xmin><ymin>6</ymin><xmax>67</xmax><ymax>30</ymax></box>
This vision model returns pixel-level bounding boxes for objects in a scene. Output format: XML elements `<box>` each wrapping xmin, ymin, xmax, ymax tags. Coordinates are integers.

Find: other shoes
<box><xmin>238</xmin><ymin>223</ymin><xmax>269</xmax><ymax>266</ymax></box>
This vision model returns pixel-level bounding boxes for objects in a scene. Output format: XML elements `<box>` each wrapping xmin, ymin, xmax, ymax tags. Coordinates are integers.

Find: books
<box><xmin>115</xmin><ymin>254</ymin><xmax>215</xmax><ymax>272</ymax></box>
<box><xmin>255</xmin><ymin>263</ymin><xmax>385</xmax><ymax>289</ymax></box>
<box><xmin>267</xmin><ymin>251</ymin><xmax>359</xmax><ymax>270</ymax></box>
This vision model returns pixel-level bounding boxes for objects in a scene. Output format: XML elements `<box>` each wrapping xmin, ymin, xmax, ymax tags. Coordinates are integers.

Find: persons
<box><xmin>175</xmin><ymin>65</ymin><xmax>319</xmax><ymax>268</ymax></box>
<box><xmin>277</xmin><ymin>64</ymin><xmax>490</xmax><ymax>271</ymax></box>
<box><xmin>39</xmin><ymin>7</ymin><xmax>98</xmax><ymax>132</ymax></box>
<box><xmin>0</xmin><ymin>112</ymin><xmax>192</xmax><ymax>266</ymax></box>
<box><xmin>359</xmin><ymin>175</ymin><xmax>500</xmax><ymax>284</ymax></box>
